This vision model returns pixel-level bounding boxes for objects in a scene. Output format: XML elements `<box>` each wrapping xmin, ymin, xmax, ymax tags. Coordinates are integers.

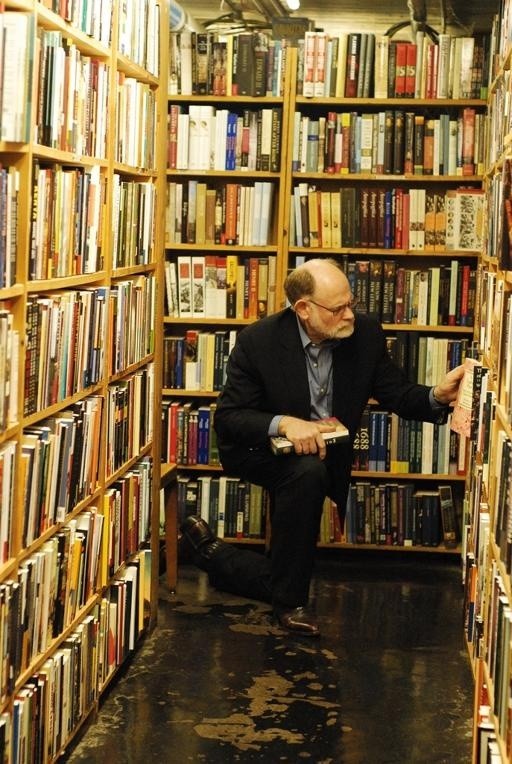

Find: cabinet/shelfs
<box><xmin>462</xmin><ymin>43</ymin><xmax>512</xmax><ymax>763</ymax></box>
<box><xmin>159</xmin><ymin>47</ymin><xmax>487</xmax><ymax>555</ymax></box>
<box><xmin>0</xmin><ymin>0</ymin><xmax>158</xmax><ymax>764</ymax></box>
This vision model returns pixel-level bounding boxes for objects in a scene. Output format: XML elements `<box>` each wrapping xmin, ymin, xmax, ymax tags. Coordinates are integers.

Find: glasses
<box><xmin>309</xmin><ymin>291</ymin><xmax>360</xmax><ymax>316</ymax></box>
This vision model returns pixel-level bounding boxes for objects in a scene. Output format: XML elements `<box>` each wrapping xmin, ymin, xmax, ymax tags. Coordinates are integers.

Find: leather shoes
<box><xmin>159</xmin><ymin>515</ymin><xmax>216</xmax><ymax>577</ymax></box>
<box><xmin>273</xmin><ymin>605</ymin><xmax>320</xmax><ymax>635</ymax></box>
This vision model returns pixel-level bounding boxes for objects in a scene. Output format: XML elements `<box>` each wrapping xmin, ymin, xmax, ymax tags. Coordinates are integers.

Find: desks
<box><xmin>160</xmin><ymin>462</ymin><xmax>180</xmax><ymax>603</ymax></box>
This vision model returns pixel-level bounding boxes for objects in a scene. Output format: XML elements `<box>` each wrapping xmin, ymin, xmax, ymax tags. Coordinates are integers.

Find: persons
<box><xmin>218</xmin><ymin>260</ymin><xmax>463</xmax><ymax>637</ymax></box>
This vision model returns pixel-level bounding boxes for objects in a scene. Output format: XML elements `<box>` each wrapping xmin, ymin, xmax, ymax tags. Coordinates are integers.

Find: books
<box><xmin>450</xmin><ymin>6</ymin><xmax>512</xmax><ymax>738</ymax></box>
<box><xmin>164</xmin><ymin>330</ymin><xmax>275</xmax><ymax>539</ymax></box>
<box><xmin>318</xmin><ymin>334</ymin><xmax>482</xmax><ymax>545</ymax></box>
<box><xmin>164</xmin><ymin>181</ymin><xmax>481</xmax><ymax>328</ymax></box>
<box><xmin>3</xmin><ymin>1</ymin><xmax>159</xmax><ymax>173</ymax></box>
<box><xmin>271</xmin><ymin>416</ymin><xmax>347</xmax><ymax>454</ymax></box>
<box><xmin>0</xmin><ymin>158</ymin><xmax>156</xmax><ymax>430</ymax></box>
<box><xmin>167</xmin><ymin>35</ymin><xmax>488</xmax><ymax>178</ymax></box>
<box><xmin>2</xmin><ymin>364</ymin><xmax>152</xmax><ymax>763</ymax></box>
